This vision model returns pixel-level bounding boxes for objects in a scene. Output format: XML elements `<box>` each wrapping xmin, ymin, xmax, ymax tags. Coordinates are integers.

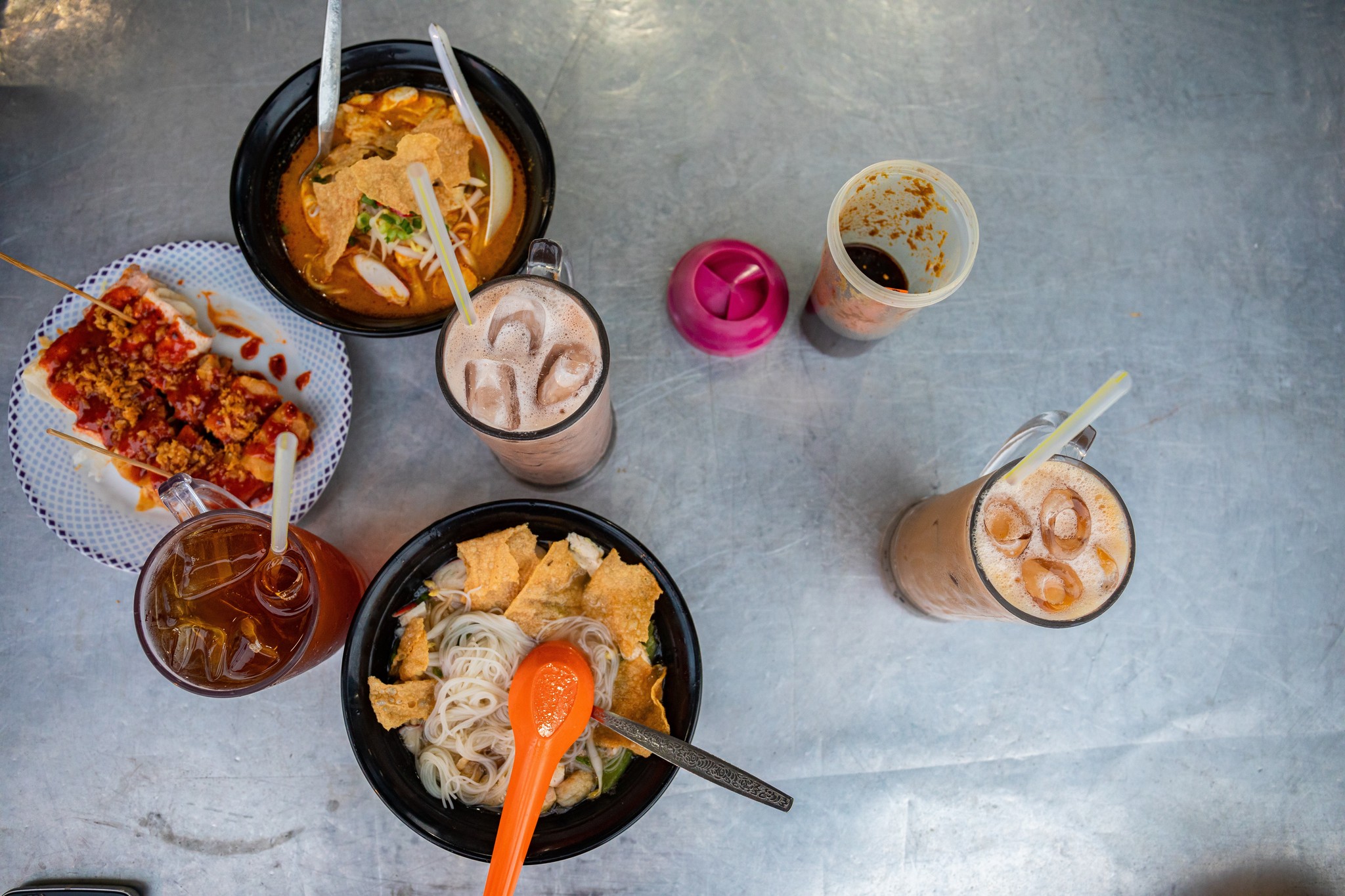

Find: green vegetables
<box><xmin>576</xmin><ymin>620</ymin><xmax>657</xmax><ymax>795</ymax></box>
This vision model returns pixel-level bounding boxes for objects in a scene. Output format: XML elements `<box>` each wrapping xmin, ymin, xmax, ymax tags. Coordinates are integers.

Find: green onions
<box><xmin>310</xmin><ymin>163</ymin><xmax>422</xmax><ymax>247</ymax></box>
